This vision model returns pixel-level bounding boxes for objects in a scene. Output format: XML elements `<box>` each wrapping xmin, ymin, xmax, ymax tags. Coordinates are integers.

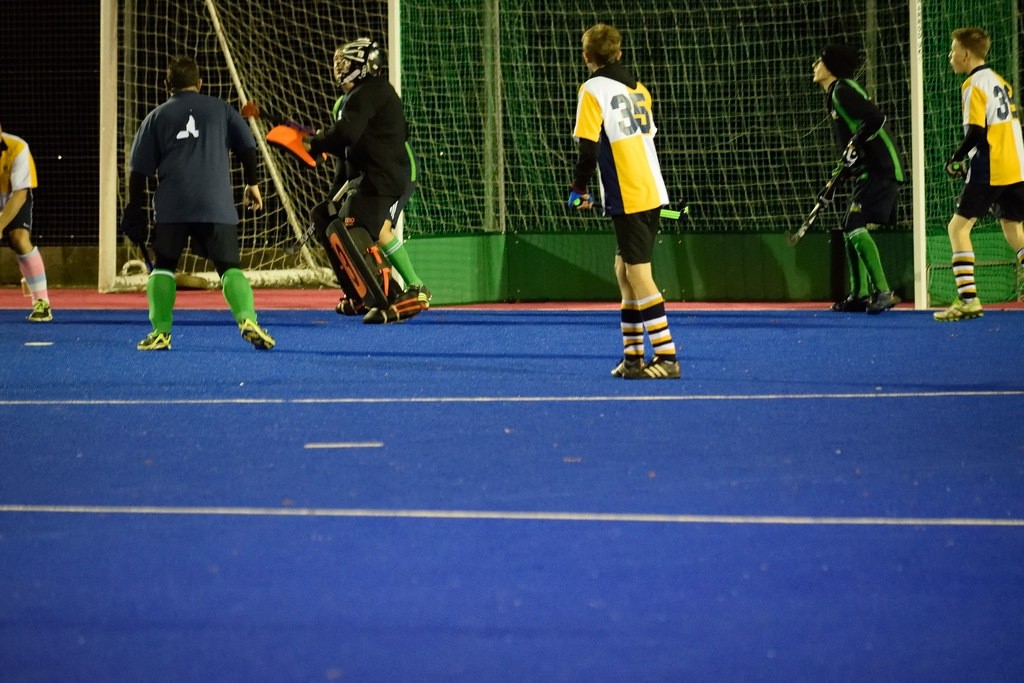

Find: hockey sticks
<box><xmin>784</xmin><ymin>175</ymin><xmax>837</xmax><ymax>248</ymax></box>
<box><xmin>567</xmin><ymin>190</ymin><xmax>691</xmax><ymax>222</ymax></box>
<box><xmin>282</xmin><ymin>179</ymin><xmax>351</xmax><ymax>257</ymax></box>
<box><xmin>237</xmin><ymin>104</ymin><xmax>316</xmax><ymax>136</ymax></box>
<box><xmin>140</xmin><ymin>242</ymin><xmax>154</xmax><ymax>274</ymax></box>
<box><xmin>953</xmin><ymin>163</ymin><xmax>969</xmax><ymax>180</ymax></box>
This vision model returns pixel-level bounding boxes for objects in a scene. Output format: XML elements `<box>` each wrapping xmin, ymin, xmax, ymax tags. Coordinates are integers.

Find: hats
<box><xmin>820</xmin><ymin>43</ymin><xmax>858</xmax><ymax>78</ymax></box>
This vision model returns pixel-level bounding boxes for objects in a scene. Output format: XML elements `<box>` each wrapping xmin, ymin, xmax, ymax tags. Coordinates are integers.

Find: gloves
<box><xmin>816</xmin><ymin>183</ymin><xmax>836</xmax><ymax>208</ymax></box>
<box><xmin>836</xmin><ymin>141</ymin><xmax>859</xmax><ymax>167</ymax></box>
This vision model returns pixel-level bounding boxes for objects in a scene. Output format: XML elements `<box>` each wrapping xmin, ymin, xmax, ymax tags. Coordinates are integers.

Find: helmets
<box><xmin>338</xmin><ymin>40</ymin><xmax>381</xmax><ymax>86</ymax></box>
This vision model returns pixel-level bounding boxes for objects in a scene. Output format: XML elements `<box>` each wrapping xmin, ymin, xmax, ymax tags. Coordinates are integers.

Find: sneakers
<box><xmin>866</xmin><ymin>290</ymin><xmax>901</xmax><ymax>315</ymax></box>
<box><xmin>622</xmin><ymin>356</ymin><xmax>680</xmax><ymax>379</ymax></box>
<box><xmin>29</xmin><ymin>299</ymin><xmax>53</xmax><ymax>322</ymax></box>
<box><xmin>934</xmin><ymin>296</ymin><xmax>984</xmax><ymax>321</ymax></box>
<box><xmin>238</xmin><ymin>319</ymin><xmax>275</xmax><ymax>349</ymax></box>
<box><xmin>137</xmin><ymin>330</ymin><xmax>171</xmax><ymax>350</ymax></box>
<box><xmin>829</xmin><ymin>293</ymin><xmax>872</xmax><ymax>313</ymax></box>
<box><xmin>611</xmin><ymin>357</ymin><xmax>645</xmax><ymax>377</ymax></box>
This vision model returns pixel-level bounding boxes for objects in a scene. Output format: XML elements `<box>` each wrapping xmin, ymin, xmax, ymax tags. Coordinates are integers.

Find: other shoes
<box><xmin>421</xmin><ymin>285</ymin><xmax>432</xmax><ymax>303</ymax></box>
<box><xmin>335</xmin><ymin>295</ymin><xmax>366</xmax><ymax>315</ymax></box>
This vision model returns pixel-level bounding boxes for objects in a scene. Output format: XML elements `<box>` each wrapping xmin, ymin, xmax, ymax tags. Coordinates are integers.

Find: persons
<box><xmin>933</xmin><ymin>29</ymin><xmax>1024</xmax><ymax>322</ymax></box>
<box><xmin>122</xmin><ymin>57</ymin><xmax>276</xmax><ymax>349</ymax></box>
<box><xmin>568</xmin><ymin>23</ymin><xmax>682</xmax><ymax>379</ymax></box>
<box><xmin>267</xmin><ymin>37</ymin><xmax>432</xmax><ymax>323</ymax></box>
<box><xmin>0</xmin><ymin>126</ymin><xmax>53</xmax><ymax>321</ymax></box>
<box><xmin>812</xmin><ymin>50</ymin><xmax>906</xmax><ymax>314</ymax></box>
